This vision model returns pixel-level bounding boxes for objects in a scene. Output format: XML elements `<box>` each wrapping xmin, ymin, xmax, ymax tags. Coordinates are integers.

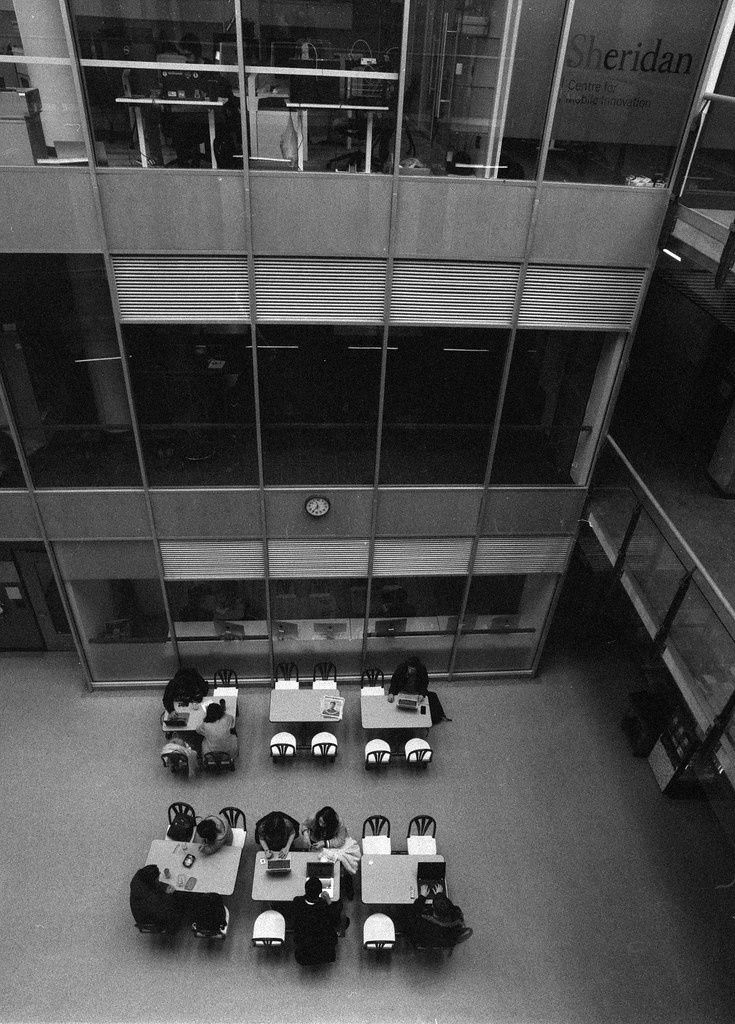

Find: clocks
<box><xmin>305</xmin><ymin>495</ymin><xmax>329</xmax><ymax>516</ymax></box>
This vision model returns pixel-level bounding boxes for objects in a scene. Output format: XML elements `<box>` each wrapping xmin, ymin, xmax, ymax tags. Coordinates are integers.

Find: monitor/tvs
<box><xmin>272</xmin><ymin>620</ymin><xmax>298</xmax><ymax>637</ymax></box>
<box><xmin>375</xmin><ymin>619</ymin><xmax>407</xmax><ymax>636</ymax></box>
<box><xmin>446</xmin><ymin>613</ymin><xmax>478</xmax><ymax>633</ymax></box>
<box><xmin>213</xmin><ymin>619</ymin><xmax>245</xmax><ymax>641</ymax></box>
<box><xmin>313</xmin><ymin>622</ymin><xmax>347</xmax><ymax>639</ymax></box>
<box><xmin>490</xmin><ymin>614</ymin><xmax>521</xmax><ymax>634</ymax></box>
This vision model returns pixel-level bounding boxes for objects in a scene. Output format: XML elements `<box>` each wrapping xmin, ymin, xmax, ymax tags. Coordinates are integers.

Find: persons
<box><xmin>196</xmin><ymin>703</ymin><xmax>239</xmax><ymax>763</ymax></box>
<box><xmin>130</xmin><ymin>865</ymin><xmax>176</xmax><ymax>928</ymax></box>
<box><xmin>301</xmin><ymin>807</ymin><xmax>356</xmax><ymax>901</ymax></box>
<box><xmin>162</xmin><ymin>669</ymin><xmax>204</xmax><ymax>719</ymax></box>
<box><xmin>292</xmin><ymin>878</ymin><xmax>343</xmax><ymax>937</ymax></box>
<box><xmin>258</xmin><ymin>814</ymin><xmax>296</xmax><ymax>859</ymax></box>
<box><xmin>411</xmin><ymin>884</ymin><xmax>463</xmax><ymax>946</ymax></box>
<box><xmin>196</xmin><ymin>815</ymin><xmax>234</xmax><ymax>856</ymax></box>
<box><xmin>162</xmin><ymin>32</ymin><xmax>242</xmax><ymax>168</ymax></box>
<box><xmin>387</xmin><ymin>657</ymin><xmax>429</xmax><ymax>704</ymax></box>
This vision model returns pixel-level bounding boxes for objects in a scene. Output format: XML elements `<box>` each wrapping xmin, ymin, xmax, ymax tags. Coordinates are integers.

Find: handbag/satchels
<box><xmin>167</xmin><ymin>813</ymin><xmax>197</xmax><ymax>842</ymax></box>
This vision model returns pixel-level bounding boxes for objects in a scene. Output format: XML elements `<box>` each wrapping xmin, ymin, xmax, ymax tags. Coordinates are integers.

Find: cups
<box><xmin>192</xmin><ymin>701</ymin><xmax>199</xmax><ymax>710</ymax></box>
<box><xmin>182</xmin><ymin>842</ymin><xmax>187</xmax><ymax>850</ymax></box>
<box><xmin>178</xmin><ymin>876</ymin><xmax>183</xmax><ymax>887</ymax></box>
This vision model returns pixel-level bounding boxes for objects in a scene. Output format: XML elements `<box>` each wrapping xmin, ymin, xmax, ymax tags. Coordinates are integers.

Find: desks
<box><xmin>116</xmin><ymin>95</ymin><xmax>229</xmax><ymax>169</ymax></box>
<box><xmin>145</xmin><ymin>839</ymin><xmax>242</xmax><ymax>896</ymax></box>
<box><xmin>285</xmin><ymin>97</ymin><xmax>389</xmax><ymax>174</ymax></box>
<box><xmin>360</xmin><ymin>695</ymin><xmax>432</xmax><ymax>729</ymax></box>
<box><xmin>249</xmin><ymin>72</ymin><xmax>308</xmax><ymax>161</ymax></box>
<box><xmin>361</xmin><ymin>855</ymin><xmax>448</xmax><ymax>905</ymax></box>
<box><xmin>162</xmin><ymin>696</ymin><xmax>238</xmax><ymax>731</ymax></box>
<box><xmin>251</xmin><ymin>851</ymin><xmax>340</xmax><ymax>903</ymax></box>
<box><xmin>270</xmin><ymin>689</ymin><xmax>343</xmax><ymax>722</ymax></box>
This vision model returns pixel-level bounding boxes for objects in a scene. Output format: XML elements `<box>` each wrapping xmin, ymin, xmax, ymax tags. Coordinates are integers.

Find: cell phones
<box><xmin>421</xmin><ymin>706</ymin><xmax>426</xmax><ymax>714</ymax></box>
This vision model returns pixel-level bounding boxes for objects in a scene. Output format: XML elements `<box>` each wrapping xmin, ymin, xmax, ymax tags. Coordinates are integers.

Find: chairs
<box><xmin>404</xmin><ymin>738</ymin><xmax>433</xmax><ymax>769</ymax></box>
<box><xmin>270</xmin><ymin>731</ymin><xmax>296</xmax><ymax>763</ymax></box>
<box><xmin>252</xmin><ymin>910</ymin><xmax>286</xmax><ymax>957</ymax></box>
<box><xmin>214</xmin><ymin>667</ymin><xmax>239</xmax><ymax>697</ymax></box>
<box><xmin>312</xmin><ymin>661</ymin><xmax>337</xmax><ymax>689</ymax></box>
<box><xmin>192</xmin><ymin>904</ymin><xmax>230</xmax><ymax>940</ymax></box>
<box><xmin>135</xmin><ymin>914</ymin><xmax>181</xmax><ymax>936</ymax></box>
<box><xmin>364</xmin><ymin>739</ymin><xmax>391</xmax><ymax>772</ymax></box>
<box><xmin>376</xmin><ymin>74</ymin><xmax>421</xmax><ymax>157</ymax></box>
<box><xmin>161</xmin><ymin>739</ymin><xmax>192</xmax><ymax>769</ymax></box>
<box><xmin>363</xmin><ymin>914</ymin><xmax>395</xmax><ymax>960</ymax></box>
<box><xmin>218</xmin><ymin>807</ymin><xmax>248</xmax><ymax>849</ymax></box>
<box><xmin>325</xmin><ymin>119</ymin><xmax>383</xmax><ymax>171</ymax></box>
<box><xmin>361</xmin><ymin>668</ymin><xmax>385</xmax><ymax>696</ymax></box>
<box><xmin>311</xmin><ymin>732</ymin><xmax>338</xmax><ymax>766</ymax></box>
<box><xmin>411</xmin><ymin>931</ymin><xmax>455</xmax><ymax>954</ymax></box>
<box><xmin>406</xmin><ymin>815</ymin><xmax>437</xmax><ymax>855</ymax></box>
<box><xmin>275</xmin><ymin>661</ymin><xmax>300</xmax><ymax>690</ymax></box>
<box><xmin>361</xmin><ymin>814</ymin><xmax>391</xmax><ymax>856</ymax></box>
<box><xmin>202</xmin><ymin>751</ymin><xmax>235</xmax><ymax>773</ymax></box>
<box><xmin>164</xmin><ymin>802</ymin><xmax>197</xmax><ymax>844</ymax></box>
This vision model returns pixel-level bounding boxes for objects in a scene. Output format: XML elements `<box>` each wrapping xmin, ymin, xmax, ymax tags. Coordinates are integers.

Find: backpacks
<box><xmin>425</xmin><ymin>689</ymin><xmax>445</xmax><ymax>724</ymax></box>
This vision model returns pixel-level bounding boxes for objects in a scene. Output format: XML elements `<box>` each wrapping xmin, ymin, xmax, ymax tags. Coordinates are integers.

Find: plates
<box><xmin>183</xmin><ymin>853</ymin><xmax>195</xmax><ymax>868</ymax></box>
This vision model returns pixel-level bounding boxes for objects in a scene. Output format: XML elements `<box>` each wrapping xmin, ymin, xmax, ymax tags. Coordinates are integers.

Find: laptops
<box><xmin>265</xmin><ymin>859</ymin><xmax>291</xmax><ymax>873</ymax></box>
<box><xmin>163</xmin><ymin>712</ymin><xmax>190</xmax><ymax>726</ymax></box>
<box><xmin>416</xmin><ymin>861</ymin><xmax>446</xmax><ymax>899</ymax></box>
<box><xmin>305</xmin><ymin>862</ymin><xmax>334</xmax><ymax>898</ymax></box>
<box><xmin>397</xmin><ymin>697</ymin><xmax>418</xmax><ymax>710</ymax></box>
